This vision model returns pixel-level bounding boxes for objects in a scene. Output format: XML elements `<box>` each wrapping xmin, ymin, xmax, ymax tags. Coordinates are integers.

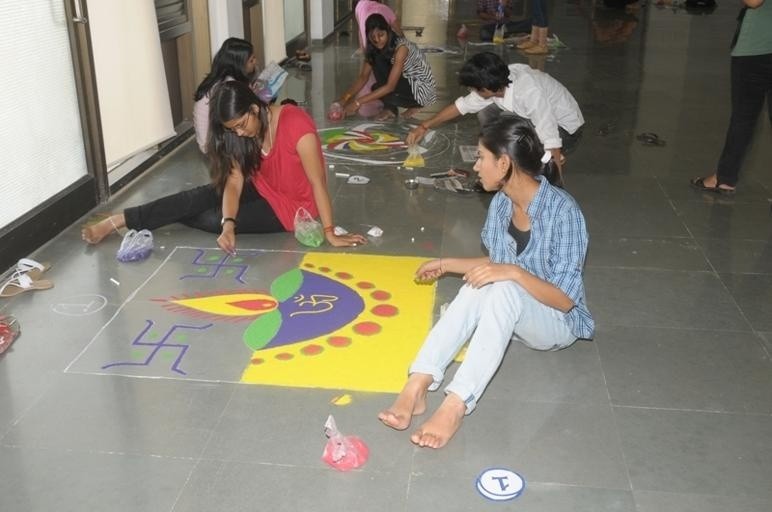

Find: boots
<box><xmin>516</xmin><ymin>25</ymin><xmax>548</xmax><ymax>54</ymax></box>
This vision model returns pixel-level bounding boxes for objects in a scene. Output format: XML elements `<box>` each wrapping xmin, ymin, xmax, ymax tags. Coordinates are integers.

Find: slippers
<box><xmin>0</xmin><ymin>257</ymin><xmax>54</xmax><ymax>298</ymax></box>
<box><xmin>636</xmin><ymin>132</ymin><xmax>664</xmax><ymax>147</ymax></box>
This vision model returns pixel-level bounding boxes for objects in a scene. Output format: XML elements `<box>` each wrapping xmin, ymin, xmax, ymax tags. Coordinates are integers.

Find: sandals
<box><xmin>287</xmin><ymin>49</ymin><xmax>312</xmax><ymax>73</ymax></box>
<box><xmin>690</xmin><ymin>174</ymin><xmax>735</xmax><ymax>196</ymax></box>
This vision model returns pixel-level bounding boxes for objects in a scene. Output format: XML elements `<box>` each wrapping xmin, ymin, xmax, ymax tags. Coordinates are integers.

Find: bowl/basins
<box><xmin>404</xmin><ymin>179</ymin><xmax>419</xmax><ymax>190</ymax></box>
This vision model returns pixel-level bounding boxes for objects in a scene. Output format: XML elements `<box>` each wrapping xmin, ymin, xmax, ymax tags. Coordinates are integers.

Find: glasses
<box><xmin>223</xmin><ymin>110</ymin><xmax>250</xmax><ymax>133</ymax></box>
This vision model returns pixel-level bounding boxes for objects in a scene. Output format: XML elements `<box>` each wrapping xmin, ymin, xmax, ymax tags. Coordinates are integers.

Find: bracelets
<box><xmin>421</xmin><ymin>121</ymin><xmax>428</xmax><ymax>133</ymax></box>
<box><xmin>354</xmin><ymin>100</ymin><xmax>361</xmax><ymax>107</ymax></box>
<box><xmin>439</xmin><ymin>257</ymin><xmax>443</xmax><ymax>272</ymax></box>
<box><xmin>218</xmin><ymin>216</ymin><xmax>239</xmax><ymax>228</ymax></box>
<box><xmin>324</xmin><ymin>226</ymin><xmax>335</xmax><ymax>234</ymax></box>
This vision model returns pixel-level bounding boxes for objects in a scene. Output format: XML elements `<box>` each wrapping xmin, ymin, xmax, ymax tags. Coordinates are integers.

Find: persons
<box><xmin>330</xmin><ymin>13</ymin><xmax>438</xmax><ymax>123</ymax></box>
<box><xmin>197</xmin><ymin>36</ymin><xmax>297</xmax><ymax>162</ymax></box>
<box><xmin>473</xmin><ymin>2</ymin><xmax>514</xmax><ymax>41</ymax></box>
<box><xmin>687</xmin><ymin>1</ymin><xmax>771</xmax><ymax>198</ymax></box>
<box><xmin>404</xmin><ymin>50</ymin><xmax>586</xmax><ymax>194</ymax></box>
<box><xmin>353</xmin><ymin>0</ymin><xmax>408</xmax><ymax>60</ymax></box>
<box><xmin>80</xmin><ymin>76</ymin><xmax>369</xmax><ymax>257</ymax></box>
<box><xmin>517</xmin><ymin>1</ymin><xmax>551</xmax><ymax>57</ymax></box>
<box><xmin>373</xmin><ymin>114</ymin><xmax>595</xmax><ymax>452</ymax></box>
